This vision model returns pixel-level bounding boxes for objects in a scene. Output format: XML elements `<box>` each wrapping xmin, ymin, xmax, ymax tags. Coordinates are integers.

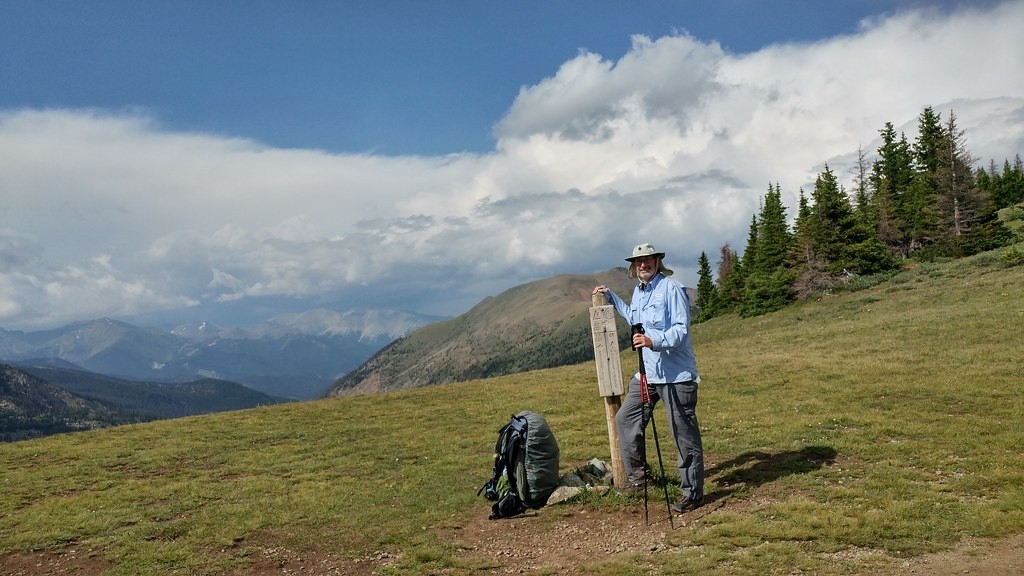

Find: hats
<box><xmin>624</xmin><ymin>243</ymin><xmax>665</xmax><ymax>262</ymax></box>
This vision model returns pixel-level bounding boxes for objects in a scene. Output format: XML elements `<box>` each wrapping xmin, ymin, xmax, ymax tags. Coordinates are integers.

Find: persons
<box><xmin>592</xmin><ymin>244</ymin><xmax>705</xmax><ymax>513</ymax></box>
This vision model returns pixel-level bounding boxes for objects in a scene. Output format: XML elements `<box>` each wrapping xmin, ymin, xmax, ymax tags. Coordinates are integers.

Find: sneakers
<box><xmin>671</xmin><ymin>496</ymin><xmax>705</xmax><ymax>513</ymax></box>
<box><xmin>616</xmin><ymin>479</ymin><xmax>654</xmax><ymax>497</ymax></box>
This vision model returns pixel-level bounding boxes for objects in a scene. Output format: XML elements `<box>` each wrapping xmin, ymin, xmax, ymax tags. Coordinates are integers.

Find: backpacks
<box><xmin>476</xmin><ymin>409</ymin><xmax>559</xmax><ymax>521</ymax></box>
